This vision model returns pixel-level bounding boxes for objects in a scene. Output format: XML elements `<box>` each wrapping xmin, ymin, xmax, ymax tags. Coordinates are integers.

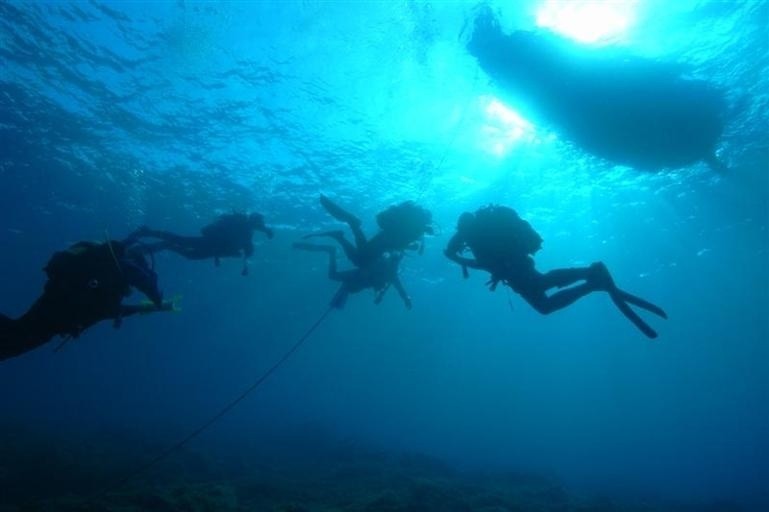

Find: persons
<box><xmin>295</xmin><ymin>231</ymin><xmax>415</xmax><ymax>311</ymax></box>
<box><xmin>306</xmin><ymin>192</ymin><xmax>433</xmax><ymax>259</ymax></box>
<box><xmin>125</xmin><ymin>212</ymin><xmax>274</xmax><ymax>276</ymax></box>
<box><xmin>0</xmin><ymin>241</ymin><xmax>164</xmax><ymax>364</ymax></box>
<box><xmin>445</xmin><ymin>208</ymin><xmax>667</xmax><ymax>337</ymax></box>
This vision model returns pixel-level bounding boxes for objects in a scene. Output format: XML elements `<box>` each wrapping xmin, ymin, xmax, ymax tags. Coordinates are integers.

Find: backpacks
<box><xmin>201</xmin><ymin>212</ymin><xmax>248</xmax><ymax>241</ymax></box>
<box><xmin>376</xmin><ymin>200</ymin><xmax>423</xmax><ymax>236</ymax></box>
<box><xmin>43</xmin><ymin>240</ymin><xmax>123</xmax><ymax>307</ymax></box>
<box><xmin>475</xmin><ymin>203</ymin><xmax>543</xmax><ymax>259</ymax></box>
